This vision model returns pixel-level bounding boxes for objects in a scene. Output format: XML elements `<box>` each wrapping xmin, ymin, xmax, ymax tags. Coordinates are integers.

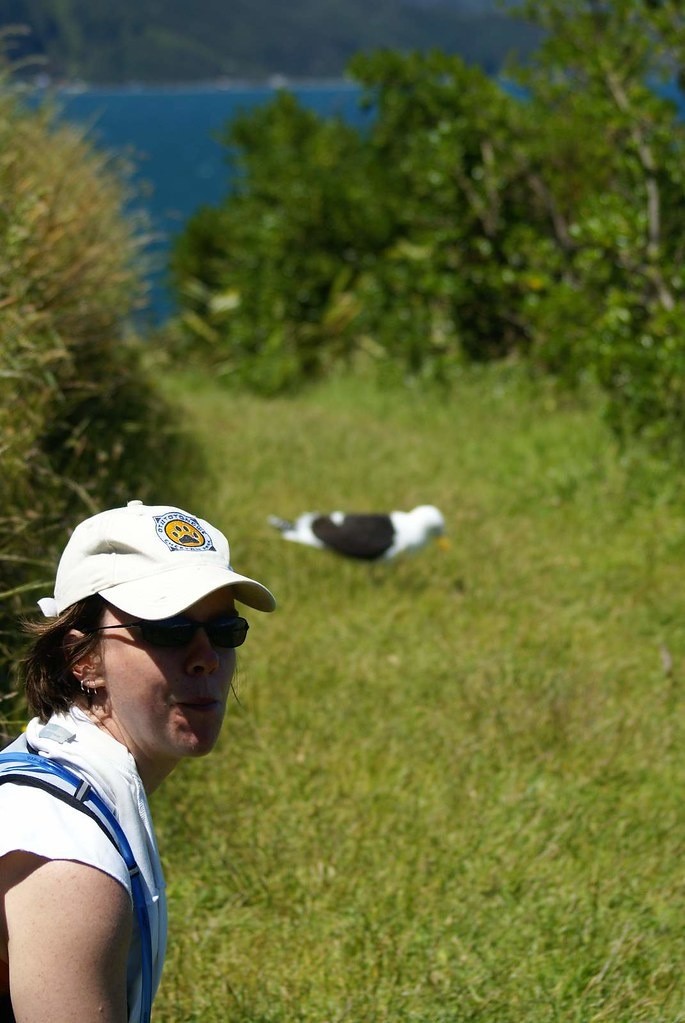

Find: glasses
<box><xmin>88</xmin><ymin>615</ymin><xmax>249</xmax><ymax>648</ymax></box>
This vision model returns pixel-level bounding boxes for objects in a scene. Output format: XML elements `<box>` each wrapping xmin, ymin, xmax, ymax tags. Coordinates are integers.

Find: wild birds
<box><xmin>268</xmin><ymin>502</ymin><xmax>445</xmax><ymax>561</ymax></box>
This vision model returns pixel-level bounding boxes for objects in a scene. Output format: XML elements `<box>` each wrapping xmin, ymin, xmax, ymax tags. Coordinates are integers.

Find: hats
<box><xmin>36</xmin><ymin>498</ymin><xmax>276</xmax><ymax>621</ymax></box>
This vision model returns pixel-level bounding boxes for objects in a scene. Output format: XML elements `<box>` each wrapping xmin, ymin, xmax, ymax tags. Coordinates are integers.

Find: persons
<box><xmin>0</xmin><ymin>500</ymin><xmax>277</xmax><ymax>1023</ymax></box>
